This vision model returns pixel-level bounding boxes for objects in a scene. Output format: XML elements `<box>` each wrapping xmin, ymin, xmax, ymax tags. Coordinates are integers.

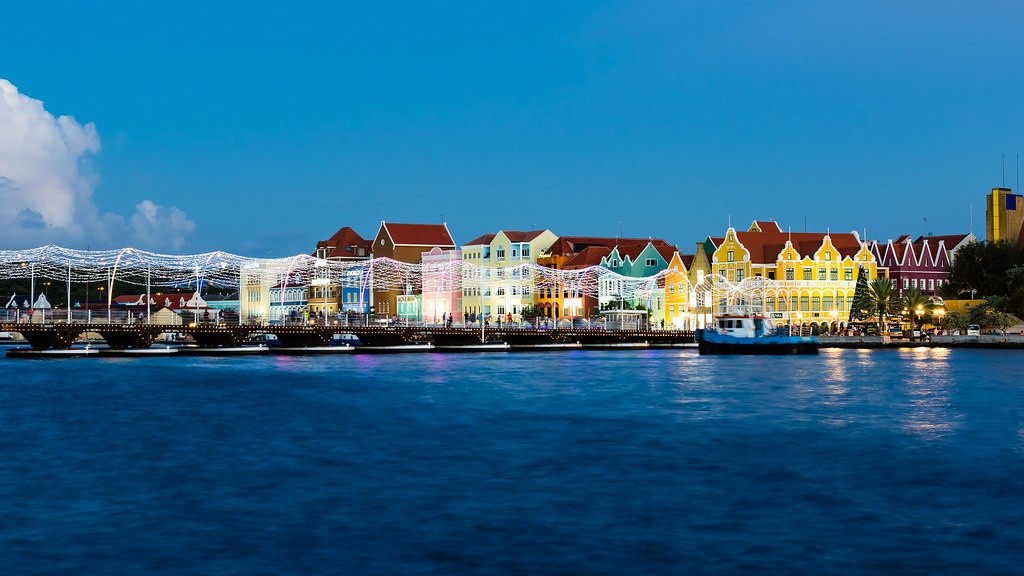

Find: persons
<box><xmin>27</xmin><ymin>307</ymin><xmax>666</xmax><ymax>330</ymax></box>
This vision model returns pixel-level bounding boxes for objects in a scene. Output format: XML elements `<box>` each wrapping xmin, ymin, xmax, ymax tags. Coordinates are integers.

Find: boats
<box><xmin>694</xmin><ymin>306</ymin><xmax>820</xmax><ymax>355</ymax></box>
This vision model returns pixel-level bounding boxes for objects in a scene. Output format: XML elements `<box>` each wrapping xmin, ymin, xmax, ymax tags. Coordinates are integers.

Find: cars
<box><xmin>863</xmin><ymin>325</ymin><xmax>981</xmax><ymax>339</ymax></box>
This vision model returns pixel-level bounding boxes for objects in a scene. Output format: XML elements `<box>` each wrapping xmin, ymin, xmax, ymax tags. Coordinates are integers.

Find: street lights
<box><xmin>901</xmin><ymin>303</ymin><xmax>946</xmax><ymax>338</ymax></box>
